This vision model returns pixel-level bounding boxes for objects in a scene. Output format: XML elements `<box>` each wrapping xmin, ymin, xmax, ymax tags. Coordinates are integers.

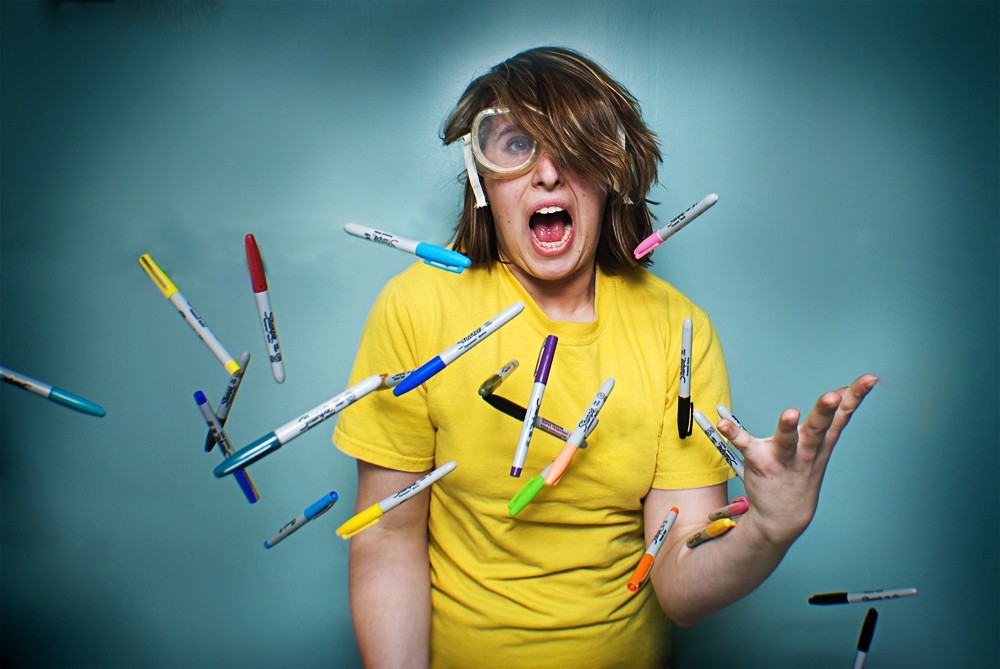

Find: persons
<box><xmin>330</xmin><ymin>47</ymin><xmax>879</xmax><ymax>669</ymax></box>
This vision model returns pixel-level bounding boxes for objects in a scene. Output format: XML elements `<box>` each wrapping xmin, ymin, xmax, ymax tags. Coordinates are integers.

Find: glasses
<box><xmin>470</xmin><ymin>107</ymin><xmax>543</xmax><ymax>179</ymax></box>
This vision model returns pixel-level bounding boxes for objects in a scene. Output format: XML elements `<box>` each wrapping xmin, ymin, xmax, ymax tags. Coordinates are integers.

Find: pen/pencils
<box><xmin>686</xmin><ymin>496</ymin><xmax>749</xmax><ymax>548</ymax></box>
<box><xmin>478</xmin><ymin>360</ymin><xmax>520</xmax><ymax>396</ymax></box>
<box><xmin>0</xmin><ymin>365</ymin><xmax>106</xmax><ymax>417</ymax></box>
<box><xmin>344</xmin><ymin>222</ymin><xmax>472</xmax><ymax>274</ymax></box>
<box><xmin>808</xmin><ymin>587</ymin><xmax>918</xmax><ymax>605</ymax></box>
<box><xmin>336</xmin><ymin>461</ymin><xmax>457</xmax><ymax>541</ymax></box>
<box><xmin>677</xmin><ymin>318</ymin><xmax>694</xmax><ymax>440</ymax></box>
<box><xmin>692</xmin><ymin>404</ymin><xmax>747</xmax><ymax>480</ymax></box>
<box><xmin>627</xmin><ymin>507</ymin><xmax>678</xmax><ymax>591</ymax></box>
<box><xmin>634</xmin><ymin>192</ymin><xmax>718</xmax><ymax>260</ymax></box>
<box><xmin>507</xmin><ymin>335</ymin><xmax>615</xmax><ymax>517</ymax></box>
<box><xmin>245</xmin><ymin>234</ymin><xmax>286</xmax><ymax>385</ymax></box>
<box><xmin>482</xmin><ymin>394</ymin><xmax>588</xmax><ymax>448</ymax></box>
<box><xmin>264</xmin><ymin>491</ymin><xmax>339</xmax><ymax>550</ymax></box>
<box><xmin>194</xmin><ymin>391</ymin><xmax>260</xmax><ymax>504</ymax></box>
<box><xmin>204</xmin><ymin>352</ymin><xmax>251</xmax><ymax>453</ymax></box>
<box><xmin>138</xmin><ymin>254</ymin><xmax>240</xmax><ymax>375</ymax></box>
<box><xmin>214</xmin><ymin>370</ymin><xmax>414</xmax><ymax>479</ymax></box>
<box><xmin>393</xmin><ymin>303</ymin><xmax>526</xmax><ymax>396</ymax></box>
<box><xmin>853</xmin><ymin>608</ymin><xmax>878</xmax><ymax>669</ymax></box>
